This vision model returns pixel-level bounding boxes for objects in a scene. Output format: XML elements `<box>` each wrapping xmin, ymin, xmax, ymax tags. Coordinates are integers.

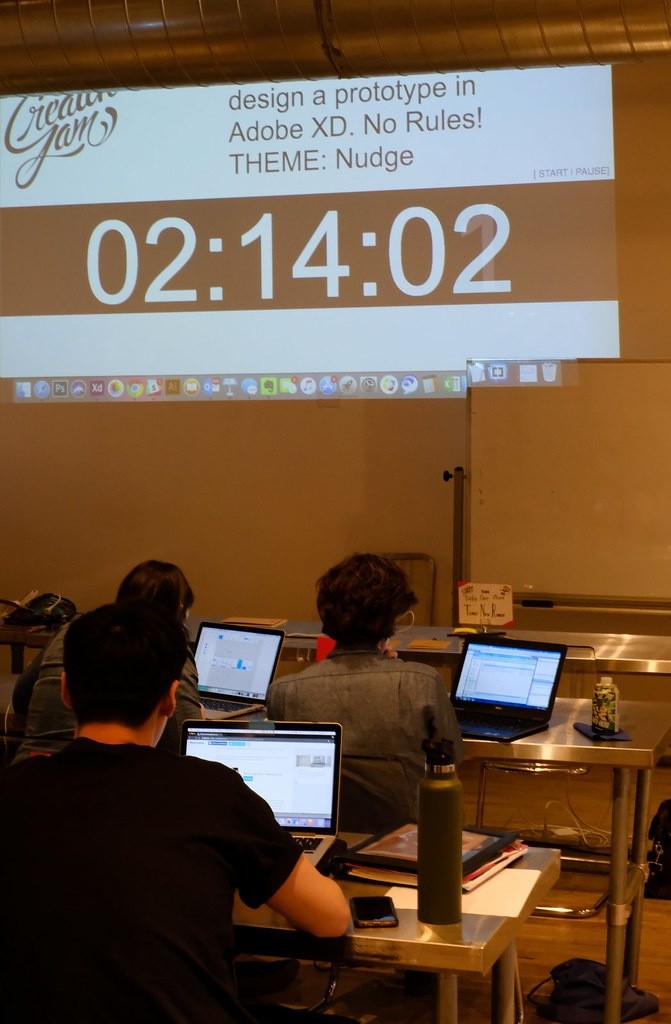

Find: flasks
<box><xmin>417</xmin><ymin>737</ymin><xmax>463</xmax><ymax>925</ymax></box>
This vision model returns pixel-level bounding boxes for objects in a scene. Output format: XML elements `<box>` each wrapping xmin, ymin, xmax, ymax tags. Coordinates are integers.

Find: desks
<box><xmin>463</xmin><ymin>697</ymin><xmax>670</xmax><ymax>1024</ymax></box>
<box><xmin>187</xmin><ymin>614</ymin><xmax>671</xmax><ymax>675</ymax></box>
<box><xmin>232</xmin><ymin>832</ymin><xmax>562</xmax><ymax>1024</ymax></box>
<box><xmin>0</xmin><ymin>625</ymin><xmax>58</xmax><ymax>675</ymax></box>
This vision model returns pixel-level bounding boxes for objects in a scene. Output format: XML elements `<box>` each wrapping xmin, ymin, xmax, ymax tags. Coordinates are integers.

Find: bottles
<box><xmin>592</xmin><ymin>677</ymin><xmax>620</xmax><ymax>735</ymax></box>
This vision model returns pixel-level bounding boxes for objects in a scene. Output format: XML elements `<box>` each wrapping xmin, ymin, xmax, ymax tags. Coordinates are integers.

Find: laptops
<box><xmin>192</xmin><ymin>620</ymin><xmax>287</xmax><ymax>722</ymax></box>
<box><xmin>448</xmin><ymin>634</ymin><xmax>568</xmax><ymax>741</ymax></box>
<box><xmin>180</xmin><ymin>718</ymin><xmax>344</xmax><ymax>870</ymax></box>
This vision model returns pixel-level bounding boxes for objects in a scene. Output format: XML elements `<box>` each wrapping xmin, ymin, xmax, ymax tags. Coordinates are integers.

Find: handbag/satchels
<box><xmin>0</xmin><ymin>593</ymin><xmax>77</xmax><ymax>626</ymax></box>
<box><xmin>527</xmin><ymin>958</ymin><xmax>659</xmax><ymax>1024</ymax></box>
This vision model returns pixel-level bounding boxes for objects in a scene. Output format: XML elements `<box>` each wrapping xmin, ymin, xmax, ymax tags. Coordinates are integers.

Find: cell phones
<box><xmin>349</xmin><ymin>896</ymin><xmax>399</xmax><ymax>928</ymax></box>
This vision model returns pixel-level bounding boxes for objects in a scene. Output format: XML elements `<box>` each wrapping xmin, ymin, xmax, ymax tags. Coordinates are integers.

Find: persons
<box><xmin>12</xmin><ymin>560</ymin><xmax>206</xmax><ymax>767</ymax></box>
<box><xmin>266</xmin><ymin>552</ymin><xmax>464</xmax><ymax>834</ymax></box>
<box><xmin>0</xmin><ymin>602</ymin><xmax>352</xmax><ymax>1024</ymax></box>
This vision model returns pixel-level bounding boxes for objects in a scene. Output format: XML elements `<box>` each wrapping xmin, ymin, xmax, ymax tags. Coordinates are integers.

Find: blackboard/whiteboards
<box><xmin>461</xmin><ymin>357</ymin><xmax>671</xmax><ymax>608</ymax></box>
